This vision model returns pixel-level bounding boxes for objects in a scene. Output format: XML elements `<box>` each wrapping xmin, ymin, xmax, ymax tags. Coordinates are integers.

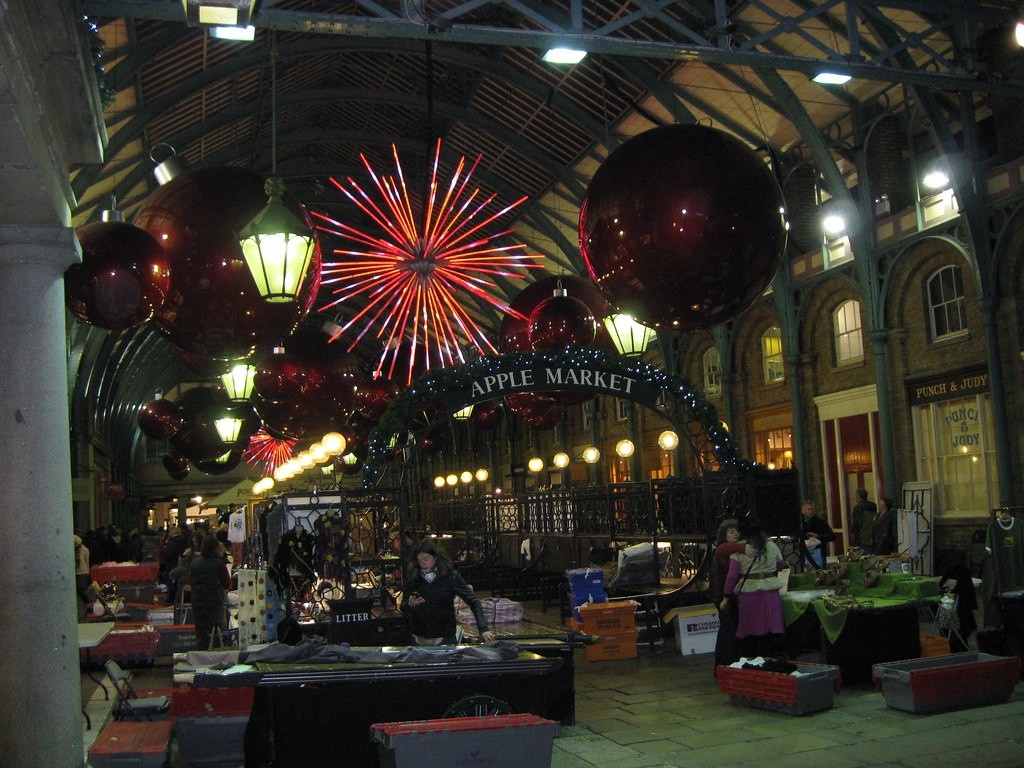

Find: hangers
<box><xmin>1000</xmin><ymin>507</ymin><xmax>1011</xmax><ymax>523</ymax></box>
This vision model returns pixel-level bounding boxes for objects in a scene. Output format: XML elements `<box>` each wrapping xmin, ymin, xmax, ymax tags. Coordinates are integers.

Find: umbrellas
<box><xmin>200</xmin><ymin>476</ymin><xmax>280</xmax><ymax>508</ymax></box>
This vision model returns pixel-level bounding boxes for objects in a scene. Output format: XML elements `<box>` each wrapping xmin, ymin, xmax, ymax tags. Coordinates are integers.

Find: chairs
<box><xmin>93</xmin><ymin>581</ymin><xmax>132</xmax><ymax>622</ymax></box>
<box><xmin>104</xmin><ymin>659</ymin><xmax>169</xmax><ymax>721</ymax></box>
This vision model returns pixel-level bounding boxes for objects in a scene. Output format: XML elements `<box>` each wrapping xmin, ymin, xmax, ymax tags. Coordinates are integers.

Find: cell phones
<box><xmin>412</xmin><ymin>592</ymin><xmax>421</xmax><ymax>599</ymax></box>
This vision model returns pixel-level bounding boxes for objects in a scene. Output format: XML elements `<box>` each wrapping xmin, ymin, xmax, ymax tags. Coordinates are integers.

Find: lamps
<box><xmin>232</xmin><ymin>29</ymin><xmax>319</xmax><ymax>304</ymax></box>
<box><xmin>599</xmin><ymin>53</ymin><xmax>652</xmax><ymax>360</ymax></box>
<box><xmin>214</xmin><ymin>355</ymin><xmax>257</xmax><ymax>463</ymax></box>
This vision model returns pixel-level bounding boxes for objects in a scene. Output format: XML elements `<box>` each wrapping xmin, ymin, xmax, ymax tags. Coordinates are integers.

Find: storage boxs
<box><xmin>873</xmin><ymin>650</ymin><xmax>1022</xmax><ymax>714</ymax></box>
<box><xmin>663</xmin><ymin>603</ymin><xmax>720</xmax><ymax>656</ymax></box>
<box><xmin>369</xmin><ymin>713</ymin><xmax>561</xmax><ymax>768</ymax></box>
<box><xmin>158</xmin><ymin>624</ymin><xmax>196</xmax><ymax>656</ymax></box>
<box><xmin>88</xmin><ymin>720</ymin><xmax>174</xmax><ymax>768</ymax></box>
<box><xmin>716</xmin><ymin>657</ymin><xmax>842</xmax><ymax>716</ymax></box>
<box><xmin>565</xmin><ymin>567</ymin><xmax>637</xmax><ymax>660</ymax></box>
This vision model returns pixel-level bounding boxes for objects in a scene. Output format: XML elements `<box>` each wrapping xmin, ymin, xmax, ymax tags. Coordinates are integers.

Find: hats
<box><xmin>169</xmin><ymin>527</ymin><xmax>182</xmax><ymax>537</ymax></box>
<box><xmin>228</xmin><ymin>503</ymin><xmax>238</xmax><ymax>509</ymax></box>
<box><xmin>74</xmin><ymin>535</ymin><xmax>83</xmax><ymax>544</ymax></box>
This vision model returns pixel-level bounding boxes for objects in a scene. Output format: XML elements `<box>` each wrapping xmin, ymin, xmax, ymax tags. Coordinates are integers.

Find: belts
<box><xmin>740</xmin><ymin>571</ymin><xmax>778</xmax><ymax>579</ymax></box>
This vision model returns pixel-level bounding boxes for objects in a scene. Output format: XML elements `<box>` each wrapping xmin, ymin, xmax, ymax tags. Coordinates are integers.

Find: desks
<box><xmin>78</xmin><ymin>622</ymin><xmax>115</xmax><ymax>730</ymax></box>
<box><xmin>91</xmin><ymin>562</ymin><xmax>159</xmax><ymax>584</ymax></box>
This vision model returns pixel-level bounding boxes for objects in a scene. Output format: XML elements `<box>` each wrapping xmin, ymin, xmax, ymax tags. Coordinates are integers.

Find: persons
<box><xmin>798</xmin><ymin>499</ymin><xmax>836</xmax><ymax>571</ymax></box>
<box><xmin>720</xmin><ymin>518</ymin><xmax>784</xmax><ymax>658</ymax></box>
<box><xmin>141</xmin><ymin>521</ymin><xmax>233</xmax><ymax>650</ymax></box>
<box><xmin>268</xmin><ymin>523</ymin><xmax>315</xmax><ymax>597</ymax></box>
<box><xmin>74</xmin><ymin>535</ymin><xmax>93</xmax><ymax>616</ymax></box>
<box><xmin>938</xmin><ymin>538</ymin><xmax>978</xmax><ymax>654</ymax></box>
<box><xmin>400</xmin><ymin>538</ymin><xmax>495</xmax><ymax>646</ymax></box>
<box><xmin>850</xmin><ymin>488</ymin><xmax>877</xmax><ymax>553</ymax></box>
<box><xmin>83</xmin><ymin>524</ymin><xmax>142</xmax><ymax>566</ymax></box>
<box><xmin>392</xmin><ymin>532</ymin><xmax>413</xmax><ymax>568</ymax></box>
<box><xmin>871</xmin><ymin>498</ymin><xmax>895</xmax><ymax>554</ymax></box>
<box><xmin>715</xmin><ymin>520</ymin><xmax>758</xmax><ymax>675</ymax></box>
<box><xmin>314</xmin><ymin>509</ymin><xmax>350</xmax><ymax>579</ymax></box>
